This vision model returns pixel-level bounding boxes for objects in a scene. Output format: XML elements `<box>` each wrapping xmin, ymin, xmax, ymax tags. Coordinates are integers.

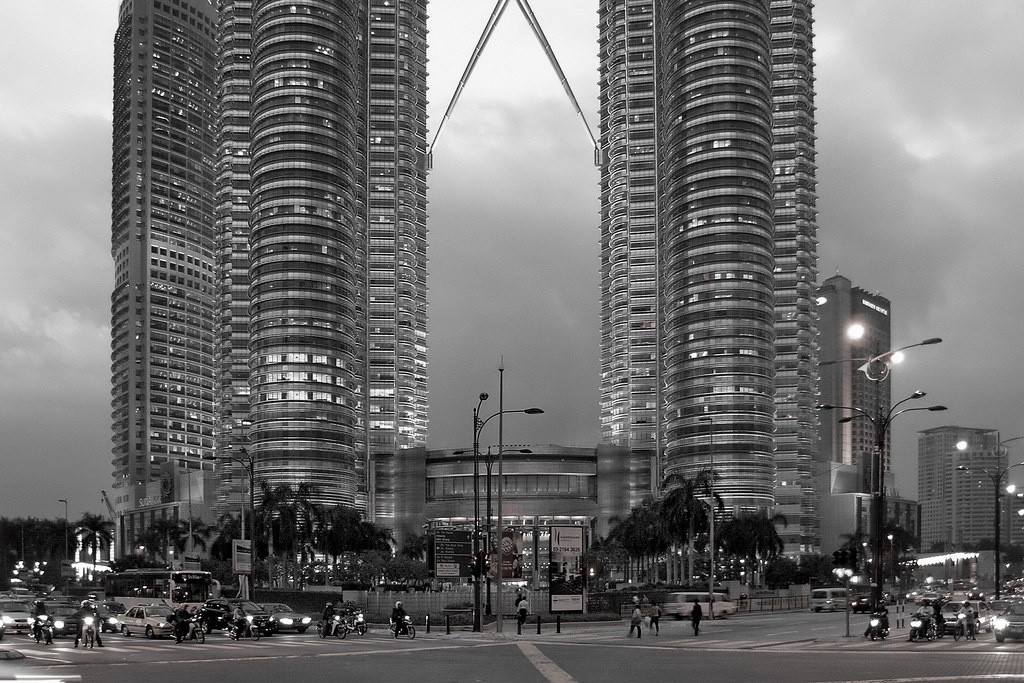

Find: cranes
<box><xmin>102</xmin><ymin>489</ymin><xmax>117</xmax><ymax>522</ymax></box>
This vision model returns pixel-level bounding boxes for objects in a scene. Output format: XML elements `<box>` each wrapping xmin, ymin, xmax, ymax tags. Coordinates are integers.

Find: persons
<box><xmin>31</xmin><ymin>599</ymin><xmax>53</xmax><ymax>645</ymax></box>
<box><xmin>341</xmin><ymin>600</ymin><xmax>360</xmax><ymax>629</ymax></box>
<box><xmin>232</xmin><ymin>603</ymin><xmax>246</xmax><ymax>642</ymax></box>
<box><xmin>322</xmin><ymin>602</ymin><xmax>338</xmax><ymax>639</ymax></box>
<box><xmin>625</xmin><ymin>593</ymin><xmax>662</xmax><ymax>639</ymax></box>
<box><xmin>516</xmin><ymin>596</ymin><xmax>531</xmax><ymax>625</ymax></box>
<box><xmin>173</xmin><ymin>602</ymin><xmax>199</xmax><ymax>644</ymax></box>
<box><xmin>905</xmin><ymin>598</ymin><xmax>941</xmax><ymax>641</ymax></box>
<box><xmin>73</xmin><ymin>600</ymin><xmax>106</xmax><ymax>649</ymax></box>
<box><xmin>690</xmin><ymin>600</ymin><xmax>703</xmax><ymax>636</ymax></box>
<box><xmin>392</xmin><ymin>601</ymin><xmax>408</xmax><ymax>639</ymax></box>
<box><xmin>861</xmin><ymin>600</ymin><xmax>889</xmax><ymax>638</ymax></box>
<box><xmin>515</xmin><ymin>593</ymin><xmax>523</xmax><ymax>607</ymax></box>
<box><xmin>954</xmin><ymin>602</ymin><xmax>976</xmax><ymax>641</ymax></box>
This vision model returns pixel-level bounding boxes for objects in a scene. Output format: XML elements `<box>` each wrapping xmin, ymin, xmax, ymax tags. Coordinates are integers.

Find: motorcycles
<box><xmin>316</xmin><ymin>608</ymin><xmax>368</xmax><ymax>640</ymax></box>
<box><xmin>28</xmin><ymin>610</ymin><xmax>56</xmax><ymax>645</ymax></box>
<box><xmin>80</xmin><ymin>613</ymin><xmax>97</xmax><ymax>648</ymax></box>
<box><xmin>165</xmin><ymin>612</ymin><xmax>205</xmax><ymax>645</ymax></box>
<box><xmin>222</xmin><ymin>612</ymin><xmax>261</xmax><ymax>641</ymax></box>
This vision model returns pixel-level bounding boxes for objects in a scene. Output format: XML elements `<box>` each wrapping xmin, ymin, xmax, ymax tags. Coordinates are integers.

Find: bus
<box><xmin>104</xmin><ymin>567</ymin><xmax>221</xmax><ymax>615</ymax></box>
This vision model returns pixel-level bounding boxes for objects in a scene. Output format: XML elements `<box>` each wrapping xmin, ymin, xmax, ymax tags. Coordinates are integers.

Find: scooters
<box><xmin>388</xmin><ymin>614</ymin><xmax>416</xmax><ymax>640</ymax></box>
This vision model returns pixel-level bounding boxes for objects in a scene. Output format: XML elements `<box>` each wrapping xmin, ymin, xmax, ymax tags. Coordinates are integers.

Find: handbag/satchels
<box><xmin>632</xmin><ymin>619</ymin><xmax>641</xmax><ymax>624</ymax></box>
<box><xmin>516</xmin><ymin>613</ymin><xmax>521</xmax><ymax>618</ymax></box>
<box><xmin>658</xmin><ymin>610</ymin><xmax>661</xmax><ymax>616</ymax></box>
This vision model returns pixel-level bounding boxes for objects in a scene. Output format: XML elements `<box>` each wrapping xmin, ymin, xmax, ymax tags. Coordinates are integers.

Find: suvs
<box><xmin>201</xmin><ymin>598</ymin><xmax>278</xmax><ymax>636</ymax></box>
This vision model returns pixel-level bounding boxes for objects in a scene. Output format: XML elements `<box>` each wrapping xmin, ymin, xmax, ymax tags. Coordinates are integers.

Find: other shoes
<box><xmin>74</xmin><ymin>645</ymin><xmax>78</xmax><ymax>647</ymax></box>
<box><xmin>906</xmin><ymin>639</ymin><xmax>912</xmax><ymax>642</ymax></box>
<box><xmin>176</xmin><ymin>640</ymin><xmax>181</xmax><ymax>644</ymax></box>
<box><xmin>972</xmin><ymin>637</ymin><xmax>976</xmax><ymax>639</ymax></box>
<box><xmin>636</xmin><ymin>636</ymin><xmax>641</xmax><ymax>638</ymax></box>
<box><xmin>50</xmin><ymin>642</ymin><xmax>54</xmax><ymax>644</ymax></box>
<box><xmin>394</xmin><ymin>634</ymin><xmax>397</xmax><ymax>640</ymax></box>
<box><xmin>320</xmin><ymin>633</ymin><xmax>325</xmax><ymax>638</ymax></box>
<box><xmin>235</xmin><ymin>636</ymin><xmax>240</xmax><ymax>641</ymax></box>
<box><xmin>655</xmin><ymin>634</ymin><xmax>658</xmax><ymax>636</ymax></box>
<box><xmin>98</xmin><ymin>644</ymin><xmax>104</xmax><ymax>647</ymax></box>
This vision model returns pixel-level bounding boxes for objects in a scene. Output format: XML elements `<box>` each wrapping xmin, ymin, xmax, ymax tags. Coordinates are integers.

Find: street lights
<box><xmin>954</xmin><ymin>431</ymin><xmax>1023</xmax><ymax>601</ymax></box>
<box><xmin>813</xmin><ymin>336</ymin><xmax>949</xmax><ymax>624</ymax></box>
<box><xmin>229</xmin><ymin>448</ymin><xmax>256</xmax><ymax>603</ymax></box>
<box><xmin>472</xmin><ymin>392</ymin><xmax>546</xmax><ymax>633</ymax></box>
<box><xmin>451</xmin><ymin>445</ymin><xmax>533</xmax><ymax>614</ymax></box>
<box><xmin>495</xmin><ymin>354</ymin><xmax>506</xmax><ymax>634</ymax></box>
<box><xmin>58</xmin><ymin>500</ymin><xmax>69</xmax><ymax>560</ymax></box>
<box><xmin>16</xmin><ymin>521</ymin><xmax>24</xmax><ymax>561</ymax></box>
<box><xmin>699</xmin><ymin>414</ymin><xmax>718</xmax><ymax>620</ymax></box>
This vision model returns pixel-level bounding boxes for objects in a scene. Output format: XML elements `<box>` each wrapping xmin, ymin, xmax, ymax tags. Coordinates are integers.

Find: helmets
<box><xmin>932</xmin><ymin>599</ymin><xmax>938</xmax><ymax>605</ymax></box>
<box><xmin>81</xmin><ymin>600</ymin><xmax>90</xmax><ymax>609</ymax></box>
<box><xmin>879</xmin><ymin>600</ymin><xmax>885</xmax><ymax>605</ymax></box>
<box><xmin>326</xmin><ymin>602</ymin><xmax>332</xmax><ymax>605</ymax></box>
<box><xmin>923</xmin><ymin>599</ymin><xmax>930</xmax><ymax>604</ymax></box>
<box><xmin>965</xmin><ymin>600</ymin><xmax>969</xmax><ymax>603</ymax></box>
<box><xmin>37</xmin><ymin>600</ymin><xmax>44</xmax><ymax>607</ymax></box>
<box><xmin>182</xmin><ymin>603</ymin><xmax>189</xmax><ymax>607</ymax></box>
<box><xmin>236</xmin><ymin>603</ymin><xmax>243</xmax><ymax>607</ymax></box>
<box><xmin>396</xmin><ymin>601</ymin><xmax>402</xmax><ymax>606</ymax></box>
<box><xmin>349</xmin><ymin>601</ymin><xmax>355</xmax><ymax>605</ymax></box>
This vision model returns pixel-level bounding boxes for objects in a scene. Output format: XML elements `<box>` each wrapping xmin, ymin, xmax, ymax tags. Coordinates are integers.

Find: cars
<box><xmin>0</xmin><ymin>586</ymin><xmax>129</xmax><ymax>634</ymax></box>
<box><xmin>810</xmin><ymin>574</ymin><xmax>1024</xmax><ymax>644</ymax></box>
<box><xmin>115</xmin><ymin>605</ymin><xmax>176</xmax><ymax>640</ymax></box>
<box><xmin>42</xmin><ymin>604</ymin><xmax>82</xmax><ymax>639</ymax></box>
<box><xmin>0</xmin><ymin>599</ymin><xmax>36</xmax><ymax>635</ymax></box>
<box><xmin>256</xmin><ymin>603</ymin><xmax>313</xmax><ymax>633</ymax></box>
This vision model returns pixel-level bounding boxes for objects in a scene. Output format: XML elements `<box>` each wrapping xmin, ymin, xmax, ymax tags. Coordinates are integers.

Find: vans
<box><xmin>666</xmin><ymin>593</ymin><xmax>737</xmax><ymax>619</ymax></box>
